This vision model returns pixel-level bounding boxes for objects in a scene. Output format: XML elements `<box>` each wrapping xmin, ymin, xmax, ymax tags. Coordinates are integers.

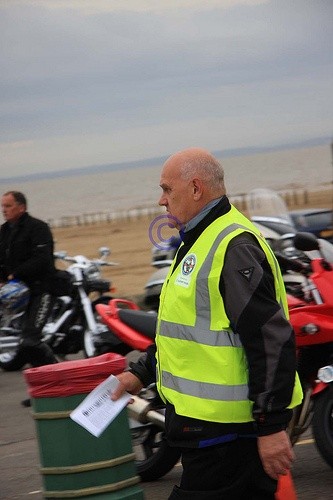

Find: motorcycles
<box><xmin>144</xmin><ymin>217</ymin><xmax>333</xmax><ymax>306</ymax></box>
<box><xmin>81</xmin><ymin>232</ymin><xmax>333</xmax><ymax>482</ymax></box>
<box><xmin>0</xmin><ymin>247</ymin><xmax>120</xmax><ymax>372</ymax></box>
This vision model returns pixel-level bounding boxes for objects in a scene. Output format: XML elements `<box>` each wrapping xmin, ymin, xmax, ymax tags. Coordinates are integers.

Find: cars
<box><xmin>289</xmin><ymin>206</ymin><xmax>333</xmax><ymax>242</ymax></box>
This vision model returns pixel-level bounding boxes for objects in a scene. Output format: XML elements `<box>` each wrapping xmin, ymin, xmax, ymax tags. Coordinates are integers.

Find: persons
<box><xmin>0</xmin><ymin>190</ymin><xmax>60</xmax><ymax>366</ymax></box>
<box><xmin>112</xmin><ymin>148</ymin><xmax>295</xmax><ymax>500</ymax></box>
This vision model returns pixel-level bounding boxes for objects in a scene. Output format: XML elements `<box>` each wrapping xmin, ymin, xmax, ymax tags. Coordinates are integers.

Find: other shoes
<box><xmin>22</xmin><ymin>398</ymin><xmax>31</xmax><ymax>407</ymax></box>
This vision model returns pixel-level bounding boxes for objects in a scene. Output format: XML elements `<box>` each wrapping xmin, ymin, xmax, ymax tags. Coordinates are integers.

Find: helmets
<box><xmin>0</xmin><ymin>280</ymin><xmax>31</xmax><ymax>315</ymax></box>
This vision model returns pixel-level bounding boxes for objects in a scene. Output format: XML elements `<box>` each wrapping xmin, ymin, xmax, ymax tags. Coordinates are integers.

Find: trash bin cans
<box><xmin>24</xmin><ymin>353</ymin><xmax>145</xmax><ymax>500</ymax></box>
